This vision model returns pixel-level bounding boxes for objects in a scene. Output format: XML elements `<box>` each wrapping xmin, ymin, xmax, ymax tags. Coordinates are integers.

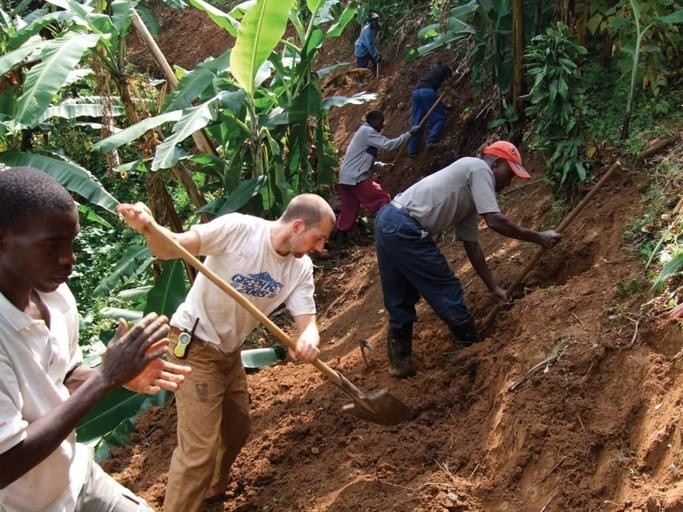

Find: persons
<box><xmin>0</xmin><ymin>163</ymin><xmax>196</xmax><ymax>512</ymax></box>
<box><xmin>114</xmin><ymin>191</ymin><xmax>337</xmax><ymax>512</ymax></box>
<box><xmin>404</xmin><ymin>56</ymin><xmax>471</xmax><ymax>158</ymax></box>
<box><xmin>331</xmin><ymin>110</ymin><xmax>424</xmax><ymax>252</ymax></box>
<box><xmin>371</xmin><ymin>136</ymin><xmax>570</xmax><ymax>382</ymax></box>
<box><xmin>351</xmin><ymin>11</ymin><xmax>383</xmax><ymax>82</ymax></box>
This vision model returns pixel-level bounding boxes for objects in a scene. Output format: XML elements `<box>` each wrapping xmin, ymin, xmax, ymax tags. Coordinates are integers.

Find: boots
<box><xmin>385</xmin><ymin>320</ymin><xmax>423</xmax><ymax>376</ymax></box>
<box><xmin>448</xmin><ymin>310</ymin><xmax>484</xmax><ymax>385</ymax></box>
<box><xmin>334</xmin><ymin>228</ymin><xmax>351</xmax><ymax>251</ymax></box>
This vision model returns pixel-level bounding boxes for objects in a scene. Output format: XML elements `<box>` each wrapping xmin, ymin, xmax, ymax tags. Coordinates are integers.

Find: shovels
<box><xmin>475</xmin><ymin>161</ymin><xmax>623</xmax><ymax>342</ymax></box>
<box><xmin>126</xmin><ymin>207</ymin><xmax>413</xmax><ymax>426</ymax></box>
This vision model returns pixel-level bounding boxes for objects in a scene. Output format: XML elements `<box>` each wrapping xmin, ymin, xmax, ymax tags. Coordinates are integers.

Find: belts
<box><xmin>389</xmin><ymin>201</ymin><xmax>432</xmax><ymax>238</ymax></box>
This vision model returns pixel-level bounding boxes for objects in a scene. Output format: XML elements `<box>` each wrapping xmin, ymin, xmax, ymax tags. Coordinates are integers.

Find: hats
<box><xmin>481</xmin><ymin>139</ymin><xmax>533</xmax><ymax>179</ymax></box>
